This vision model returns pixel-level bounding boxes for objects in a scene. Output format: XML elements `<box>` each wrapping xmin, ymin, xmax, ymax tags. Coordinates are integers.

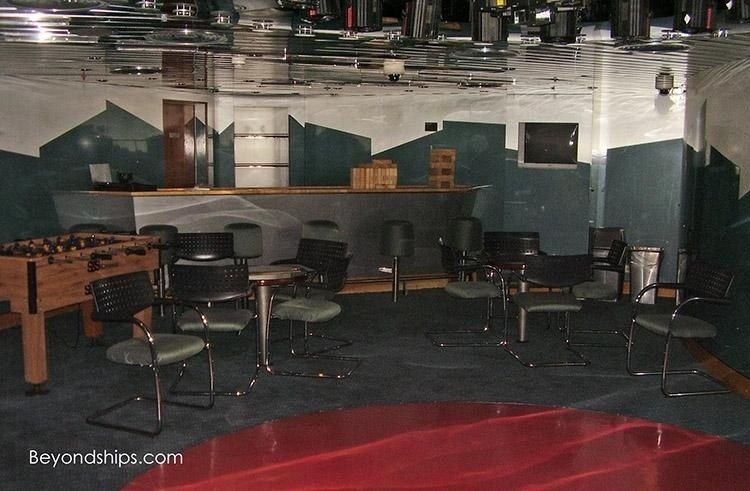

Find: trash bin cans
<box><xmin>627</xmin><ymin>244</ymin><xmax>664</xmax><ymax>304</ymax></box>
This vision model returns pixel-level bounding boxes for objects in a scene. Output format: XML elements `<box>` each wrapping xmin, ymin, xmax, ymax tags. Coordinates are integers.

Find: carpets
<box><xmin>117</xmin><ymin>397</ymin><xmax>748</xmax><ymax>491</ymax></box>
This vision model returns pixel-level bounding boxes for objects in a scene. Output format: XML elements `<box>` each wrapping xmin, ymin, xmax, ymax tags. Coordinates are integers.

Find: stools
<box><xmin>138</xmin><ymin>213</ymin><xmax>488</xmax><ymax>303</ymax></box>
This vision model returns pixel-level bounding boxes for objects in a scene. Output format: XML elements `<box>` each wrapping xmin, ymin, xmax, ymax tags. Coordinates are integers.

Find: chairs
<box><xmin>85</xmin><ymin>229</ymin><xmax>360</xmax><ymax>437</ymax></box>
<box><xmin>424</xmin><ymin>225</ymin><xmax>739</xmax><ymax>400</ymax></box>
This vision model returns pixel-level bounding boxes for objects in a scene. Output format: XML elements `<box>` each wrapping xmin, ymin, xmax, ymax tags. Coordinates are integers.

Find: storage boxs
<box><xmin>348</xmin><ymin>156</ymin><xmax>401</xmax><ymax>192</ymax></box>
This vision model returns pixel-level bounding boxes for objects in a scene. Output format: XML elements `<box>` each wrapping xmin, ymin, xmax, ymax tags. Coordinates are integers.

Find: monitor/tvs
<box><xmin>522</xmin><ymin>121</ymin><xmax>579</xmax><ymax>165</ymax></box>
<box><xmin>89</xmin><ymin>163</ymin><xmax>113</xmax><ymax>185</ymax></box>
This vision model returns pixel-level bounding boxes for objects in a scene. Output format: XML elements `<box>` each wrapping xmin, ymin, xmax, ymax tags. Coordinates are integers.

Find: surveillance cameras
<box><xmin>384</xmin><ymin>60</ymin><xmax>405</xmax><ymax>80</ymax></box>
<box><xmin>656</xmin><ymin>74</ymin><xmax>674</xmax><ymax>95</ymax></box>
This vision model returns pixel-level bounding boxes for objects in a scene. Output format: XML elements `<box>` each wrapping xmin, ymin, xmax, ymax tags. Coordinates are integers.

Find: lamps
<box><xmin>654</xmin><ymin>71</ymin><xmax>681</xmax><ymax>98</ymax></box>
<box><xmin>379</xmin><ymin>53</ymin><xmax>410</xmax><ymax>83</ymax></box>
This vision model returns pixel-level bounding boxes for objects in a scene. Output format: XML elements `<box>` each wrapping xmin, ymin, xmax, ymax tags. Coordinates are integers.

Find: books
<box><xmin>430</xmin><ymin>147</ymin><xmax>455</xmax><ymax>190</ymax></box>
<box><xmin>351</xmin><ymin>158</ymin><xmax>398</xmax><ymax>190</ymax></box>
